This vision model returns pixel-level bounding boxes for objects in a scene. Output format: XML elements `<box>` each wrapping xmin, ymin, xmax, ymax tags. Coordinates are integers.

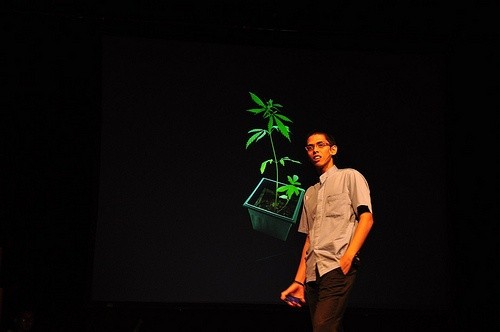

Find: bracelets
<box><xmin>294</xmin><ymin>279</ymin><xmax>306</xmax><ymax>287</ymax></box>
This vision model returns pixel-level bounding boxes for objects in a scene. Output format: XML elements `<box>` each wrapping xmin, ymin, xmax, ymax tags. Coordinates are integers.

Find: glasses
<box><xmin>305</xmin><ymin>141</ymin><xmax>331</xmax><ymax>151</ymax></box>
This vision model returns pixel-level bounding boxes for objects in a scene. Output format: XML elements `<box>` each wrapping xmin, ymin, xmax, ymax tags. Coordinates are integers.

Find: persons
<box><xmin>280</xmin><ymin>130</ymin><xmax>374</xmax><ymax>332</ymax></box>
